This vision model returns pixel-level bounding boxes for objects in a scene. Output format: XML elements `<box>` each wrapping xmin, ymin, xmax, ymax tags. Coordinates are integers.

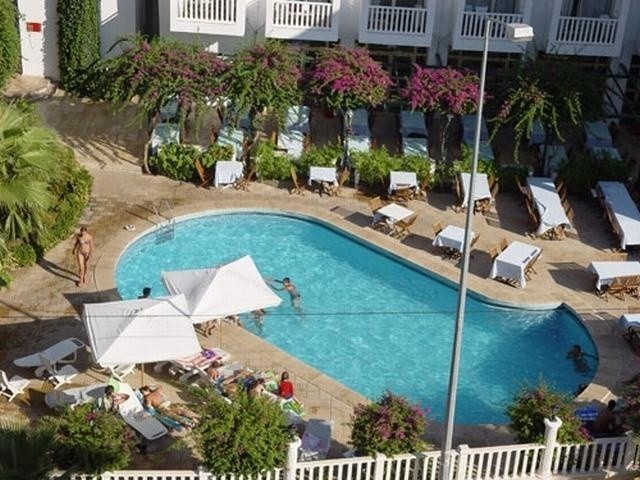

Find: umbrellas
<box><xmin>82</xmin><ymin>293</ymin><xmax>201</xmax><ymax>387</ymax></box>
<box><xmin>160</xmin><ymin>255</ymin><xmax>284</xmax><ymax>349</ymax></box>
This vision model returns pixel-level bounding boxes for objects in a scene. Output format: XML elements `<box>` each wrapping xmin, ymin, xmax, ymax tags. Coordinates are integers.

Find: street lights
<box><xmin>440</xmin><ymin>15</ymin><xmax>534</xmax><ymax>475</ymax></box>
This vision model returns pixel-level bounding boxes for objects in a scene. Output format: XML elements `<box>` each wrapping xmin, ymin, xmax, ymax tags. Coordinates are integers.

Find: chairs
<box><xmin>627</xmin><ymin>275</ymin><xmax>639</xmax><ymax>299</ymax></box>
<box><xmin>601</xmin><ymin>277</ymin><xmax>629</xmax><ymax>302</ymax></box>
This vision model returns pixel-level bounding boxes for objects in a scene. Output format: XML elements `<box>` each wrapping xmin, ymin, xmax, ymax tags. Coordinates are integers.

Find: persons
<box><xmin>94</xmin><ymin>385</ymin><xmax>129</xmax><ymax>416</ymax></box>
<box><xmin>591</xmin><ymin>400</ymin><xmax>618</xmax><ymax>435</ymax></box>
<box><xmin>177</xmin><ymin>347</ymin><xmax>294</xmax><ymax>404</ymax></box>
<box><xmin>139</xmin><ymin>384</ymin><xmax>202</xmax><ymax>432</ymax></box>
<box><xmin>198</xmin><ymin>308</ymin><xmax>265</xmax><ymax>338</ymax></box>
<box><xmin>264</xmin><ymin>275</ymin><xmax>300</xmax><ymax>306</ymax></box>
<box><xmin>137</xmin><ymin>288</ymin><xmax>151</xmax><ymax>299</ymax></box>
<box><xmin>568</xmin><ymin>343</ymin><xmax>599</xmax><ymax>373</ymax></box>
<box><xmin>73</xmin><ymin>227</ymin><xmax>94</xmax><ymax>287</ymax></box>
<box><xmin>147</xmin><ymin>106</ymin><xmax>160</xmax><ymax>134</ymax></box>
<box><xmin>579</xmin><ymin>384</ymin><xmax>587</xmax><ymax>394</ymax></box>
